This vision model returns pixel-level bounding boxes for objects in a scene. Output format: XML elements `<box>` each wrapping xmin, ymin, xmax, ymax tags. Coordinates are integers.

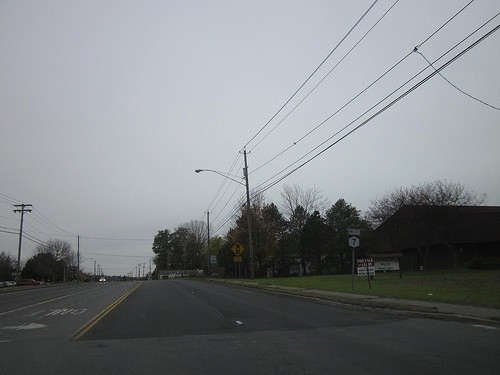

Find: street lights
<box><xmin>195</xmin><ymin>169</ymin><xmax>255</xmax><ymax>281</ymax></box>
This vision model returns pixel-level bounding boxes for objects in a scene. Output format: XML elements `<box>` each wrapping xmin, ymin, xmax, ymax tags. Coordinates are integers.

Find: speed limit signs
<box><xmin>348</xmin><ymin>229</ymin><xmax>361</xmax><ymax>249</ymax></box>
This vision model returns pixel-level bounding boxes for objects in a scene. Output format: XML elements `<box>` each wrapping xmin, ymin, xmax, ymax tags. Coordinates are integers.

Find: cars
<box><xmin>100</xmin><ymin>277</ymin><xmax>106</xmax><ymax>281</ymax></box>
<box><xmin>17</xmin><ymin>279</ymin><xmax>45</xmax><ymax>285</ymax></box>
